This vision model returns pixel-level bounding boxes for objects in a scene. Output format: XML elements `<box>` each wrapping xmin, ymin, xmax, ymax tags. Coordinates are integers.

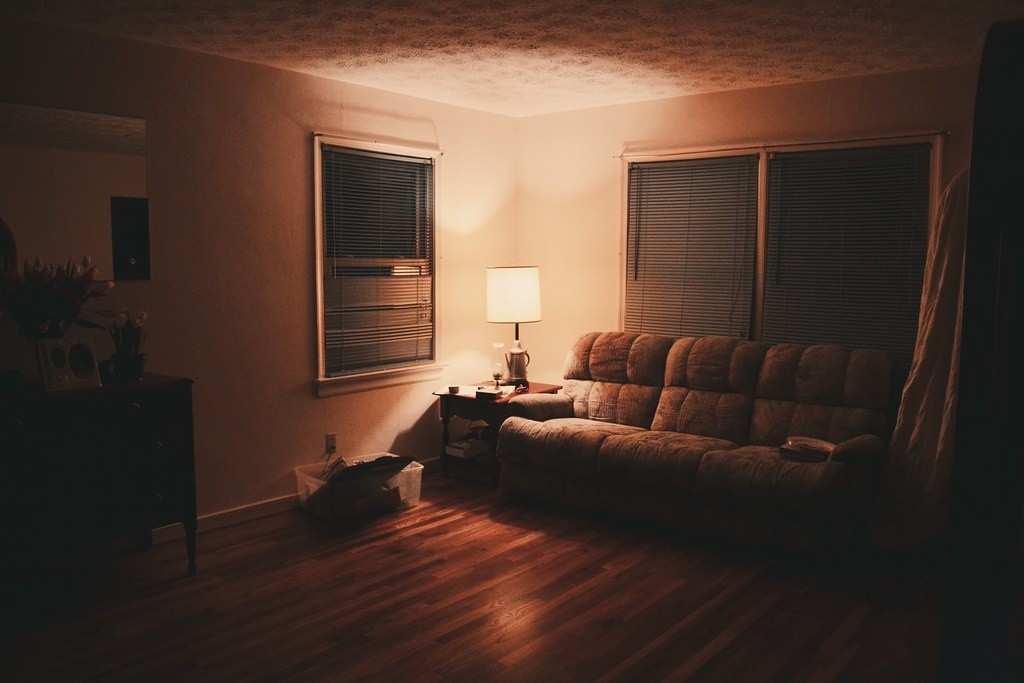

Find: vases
<box><xmin>112</xmin><ymin>354</ymin><xmax>146</xmax><ymax>384</ymax></box>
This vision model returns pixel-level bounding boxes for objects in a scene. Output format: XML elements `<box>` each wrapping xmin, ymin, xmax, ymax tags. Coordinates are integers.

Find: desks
<box><xmin>0</xmin><ymin>370</ymin><xmax>197</xmax><ymax>577</ymax></box>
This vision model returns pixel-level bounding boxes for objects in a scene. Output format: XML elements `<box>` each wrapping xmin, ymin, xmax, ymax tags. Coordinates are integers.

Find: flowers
<box><xmin>109</xmin><ymin>308</ymin><xmax>151</xmax><ymax>355</ymax></box>
<box><xmin>0</xmin><ymin>254</ymin><xmax>116</xmax><ymax>340</ymax></box>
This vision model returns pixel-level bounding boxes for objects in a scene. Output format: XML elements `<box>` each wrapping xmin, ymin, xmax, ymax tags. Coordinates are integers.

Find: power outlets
<box><xmin>326</xmin><ymin>432</ymin><xmax>336</xmax><ymax>453</ymax></box>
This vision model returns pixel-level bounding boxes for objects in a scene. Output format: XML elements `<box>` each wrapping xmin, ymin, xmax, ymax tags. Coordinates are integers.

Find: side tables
<box><xmin>432</xmin><ymin>378</ymin><xmax>563</xmax><ymax>488</ymax></box>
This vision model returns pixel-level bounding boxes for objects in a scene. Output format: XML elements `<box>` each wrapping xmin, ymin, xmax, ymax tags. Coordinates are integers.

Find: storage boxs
<box><xmin>295</xmin><ymin>452</ymin><xmax>425</xmax><ymax>519</ymax></box>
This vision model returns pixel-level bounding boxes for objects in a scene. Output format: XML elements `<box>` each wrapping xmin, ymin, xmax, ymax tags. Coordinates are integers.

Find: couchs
<box><xmin>498</xmin><ymin>332</ymin><xmax>891</xmax><ymax>567</ymax></box>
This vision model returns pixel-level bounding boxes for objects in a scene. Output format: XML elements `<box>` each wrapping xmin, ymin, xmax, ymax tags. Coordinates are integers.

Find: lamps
<box><xmin>487</xmin><ymin>265</ymin><xmax>542</xmax><ymax>389</ymax></box>
